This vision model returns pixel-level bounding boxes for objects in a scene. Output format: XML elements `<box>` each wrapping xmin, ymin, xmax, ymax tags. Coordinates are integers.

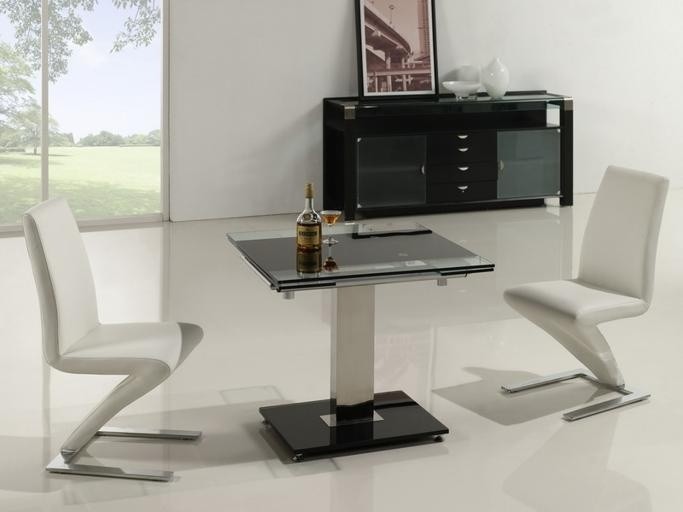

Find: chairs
<box><xmin>23</xmin><ymin>196</ymin><xmax>204</xmax><ymax>483</ymax></box>
<box><xmin>500</xmin><ymin>165</ymin><xmax>669</xmax><ymax>421</ymax></box>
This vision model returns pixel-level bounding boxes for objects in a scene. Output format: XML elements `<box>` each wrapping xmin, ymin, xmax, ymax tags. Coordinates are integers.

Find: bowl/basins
<box><xmin>442</xmin><ymin>81</ymin><xmax>481</xmax><ymax>97</ymax></box>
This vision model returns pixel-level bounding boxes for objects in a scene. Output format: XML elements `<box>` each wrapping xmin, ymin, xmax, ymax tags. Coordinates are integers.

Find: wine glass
<box><xmin>319</xmin><ymin>210</ymin><xmax>342</xmax><ymax>245</ymax></box>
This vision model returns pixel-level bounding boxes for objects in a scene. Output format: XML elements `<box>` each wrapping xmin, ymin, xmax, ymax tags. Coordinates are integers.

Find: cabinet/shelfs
<box><xmin>324</xmin><ymin>90</ymin><xmax>572</xmax><ymax>221</ymax></box>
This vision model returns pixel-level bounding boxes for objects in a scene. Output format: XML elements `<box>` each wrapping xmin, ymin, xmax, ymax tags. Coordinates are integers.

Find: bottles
<box><xmin>296</xmin><ymin>183</ymin><xmax>323</xmax><ymax>274</ymax></box>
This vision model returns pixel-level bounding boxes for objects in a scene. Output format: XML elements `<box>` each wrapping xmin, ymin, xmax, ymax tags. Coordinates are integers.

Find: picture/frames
<box><xmin>355</xmin><ymin>0</ymin><xmax>441</xmax><ymax>99</ymax></box>
